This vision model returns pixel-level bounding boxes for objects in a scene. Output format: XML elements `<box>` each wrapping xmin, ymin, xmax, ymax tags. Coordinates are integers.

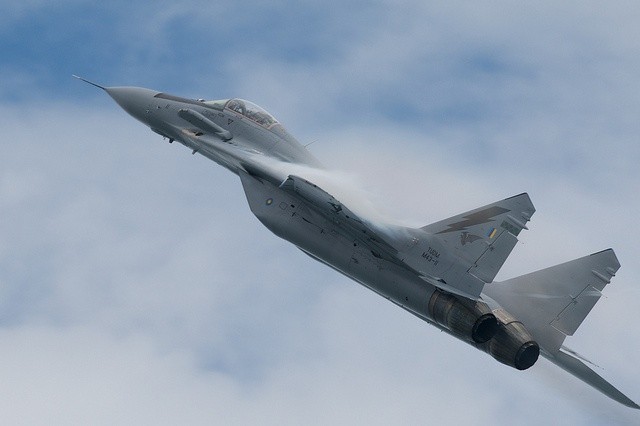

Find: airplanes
<box><xmin>72</xmin><ymin>72</ymin><xmax>640</xmax><ymax>410</ymax></box>
